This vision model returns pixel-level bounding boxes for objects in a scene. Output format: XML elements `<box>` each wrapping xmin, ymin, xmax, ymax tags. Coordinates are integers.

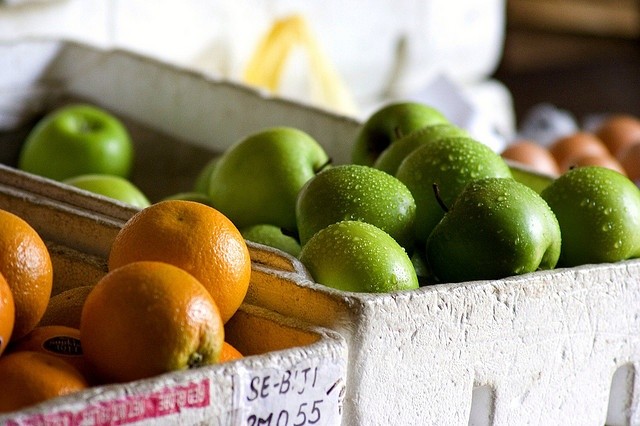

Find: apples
<box><xmin>426</xmin><ymin>178</ymin><xmax>562</xmax><ymax>282</ymax></box>
<box><xmin>66</xmin><ymin>174</ymin><xmax>150</xmax><ymax>209</ymax></box>
<box><xmin>242</xmin><ymin>224</ymin><xmax>300</xmax><ymax>255</ymax></box>
<box><xmin>397</xmin><ymin>137</ymin><xmax>514</xmax><ymax>242</ymax></box>
<box><xmin>160</xmin><ymin>192</ymin><xmax>209</xmax><ymax>206</ymax></box>
<box><xmin>18</xmin><ymin>103</ymin><xmax>131</xmax><ymax>182</ymax></box>
<box><xmin>193</xmin><ymin>157</ymin><xmax>218</xmax><ymax>191</ymax></box>
<box><xmin>350</xmin><ymin>102</ymin><xmax>447</xmax><ymax>165</ymax></box>
<box><xmin>211</xmin><ymin>127</ymin><xmax>332</xmax><ymax>231</ymax></box>
<box><xmin>294</xmin><ymin>165</ymin><xmax>417</xmax><ymax>250</ymax></box>
<box><xmin>372</xmin><ymin>122</ymin><xmax>463</xmax><ymax>176</ymax></box>
<box><xmin>540</xmin><ymin>166</ymin><xmax>639</xmax><ymax>259</ymax></box>
<box><xmin>298</xmin><ymin>221</ymin><xmax>419</xmax><ymax>292</ymax></box>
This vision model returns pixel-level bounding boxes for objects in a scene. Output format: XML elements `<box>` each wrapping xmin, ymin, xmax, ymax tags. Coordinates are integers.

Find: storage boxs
<box><xmin>0</xmin><ymin>244</ymin><xmax>350</xmax><ymax>426</ymax></box>
<box><xmin>1</xmin><ymin>44</ymin><xmax>640</xmax><ymax>425</ymax></box>
